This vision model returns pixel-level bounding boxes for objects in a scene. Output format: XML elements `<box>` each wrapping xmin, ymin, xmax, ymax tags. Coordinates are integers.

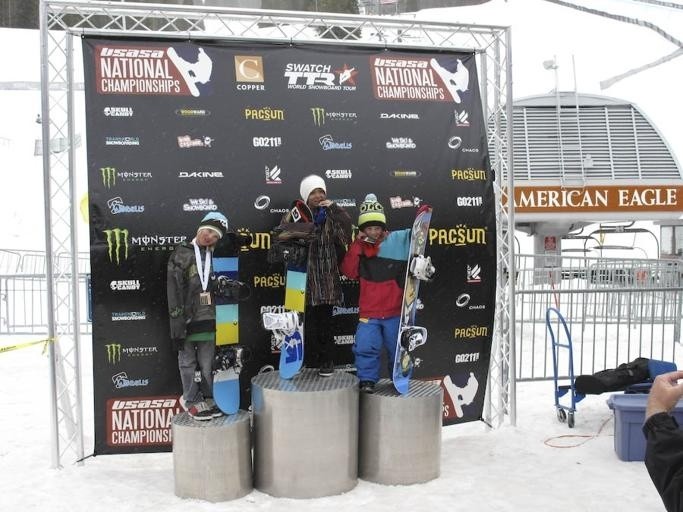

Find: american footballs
<box><xmin>212</xmin><ymin>233</ymin><xmax>241</xmax><ymax>415</ymax></box>
<box><xmin>278</xmin><ymin>200</ymin><xmax>314</xmax><ymax>380</ymax></box>
<box><xmin>394</xmin><ymin>204</ymin><xmax>433</xmax><ymax>394</ymax></box>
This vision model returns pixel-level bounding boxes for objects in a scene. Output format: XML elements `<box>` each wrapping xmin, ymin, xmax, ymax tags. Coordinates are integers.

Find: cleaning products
<box><xmin>546</xmin><ymin>306</ymin><xmax>654</xmax><ymax>427</ymax></box>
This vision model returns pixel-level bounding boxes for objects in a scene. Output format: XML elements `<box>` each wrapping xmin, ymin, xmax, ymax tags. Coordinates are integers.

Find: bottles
<box><xmin>356</xmin><ymin>192</ymin><xmax>388</xmax><ymax>229</ymax></box>
<box><xmin>299</xmin><ymin>174</ymin><xmax>328</xmax><ymax>207</ymax></box>
<box><xmin>196</xmin><ymin>211</ymin><xmax>230</xmax><ymax>240</ymax></box>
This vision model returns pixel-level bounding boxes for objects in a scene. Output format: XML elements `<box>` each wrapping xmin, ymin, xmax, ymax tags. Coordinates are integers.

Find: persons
<box><xmin>167</xmin><ymin>212</ymin><xmax>252</xmax><ymax>418</ymax></box>
<box><xmin>641</xmin><ymin>371</ymin><xmax>683</xmax><ymax>512</ymax></box>
<box><xmin>339</xmin><ymin>193</ymin><xmax>433</xmax><ymax>393</ymax></box>
<box><xmin>271</xmin><ymin>175</ymin><xmax>352</xmax><ymax>376</ymax></box>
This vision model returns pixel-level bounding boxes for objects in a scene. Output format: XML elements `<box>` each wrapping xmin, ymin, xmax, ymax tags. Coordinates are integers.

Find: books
<box><xmin>607</xmin><ymin>391</ymin><xmax>683</xmax><ymax>462</ymax></box>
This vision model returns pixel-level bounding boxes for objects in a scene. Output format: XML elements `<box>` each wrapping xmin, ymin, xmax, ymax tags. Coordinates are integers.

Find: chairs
<box><xmin>317</xmin><ymin>359</ymin><xmax>335</xmax><ymax>378</ymax></box>
<box><xmin>203</xmin><ymin>397</ymin><xmax>224</xmax><ymax>418</ymax></box>
<box><xmin>176</xmin><ymin>393</ymin><xmax>213</xmax><ymax>421</ymax></box>
<box><xmin>359</xmin><ymin>379</ymin><xmax>376</xmax><ymax>395</ymax></box>
